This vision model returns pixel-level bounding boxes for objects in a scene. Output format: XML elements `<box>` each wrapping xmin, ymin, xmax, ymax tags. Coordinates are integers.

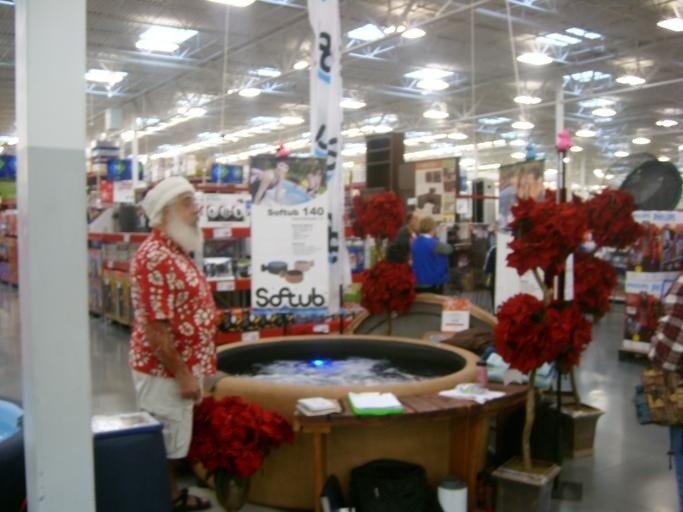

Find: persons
<box><xmin>500</xmin><ymin>162</ymin><xmax>547</xmax><ymax>224</ymax></box>
<box><xmin>306</xmin><ymin>167</ymin><xmax>323</xmax><ymax>200</ymax></box>
<box><xmin>482</xmin><ymin>244</ymin><xmax>496</xmax><ymax>308</ymax></box>
<box><xmin>394</xmin><ymin>211</ymin><xmax>422</xmax><ymax>255</ymax></box>
<box><xmin>249</xmin><ymin>156</ymin><xmax>291</xmax><ymax>205</ymax></box>
<box><xmin>129</xmin><ymin>175</ymin><xmax>217</xmax><ymax>512</ymax></box>
<box><xmin>447</xmin><ymin>225</ymin><xmax>462</xmax><ymax>245</ymax></box>
<box><xmin>649</xmin><ymin>270</ymin><xmax>683</xmax><ymax>512</ymax></box>
<box><xmin>411</xmin><ymin>216</ymin><xmax>454</xmax><ymax>295</ymax></box>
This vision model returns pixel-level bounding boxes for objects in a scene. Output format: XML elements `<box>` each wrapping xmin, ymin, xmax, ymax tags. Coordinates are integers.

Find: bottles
<box><xmin>435</xmin><ymin>473</ymin><xmax>469</xmax><ymax>512</ymax></box>
<box><xmin>343</xmin><ymin>234</ymin><xmax>364</xmax><ymax>272</ymax></box>
<box><xmin>142</xmin><ymin>150</ymin><xmax>198</xmax><ymax>184</ymax></box>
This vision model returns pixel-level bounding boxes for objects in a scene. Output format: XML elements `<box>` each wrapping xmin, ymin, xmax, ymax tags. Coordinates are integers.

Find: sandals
<box><xmin>170</xmin><ymin>486</ymin><xmax>213</xmax><ymax>512</ymax></box>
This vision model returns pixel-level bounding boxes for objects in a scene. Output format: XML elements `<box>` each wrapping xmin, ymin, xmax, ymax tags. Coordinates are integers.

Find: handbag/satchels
<box><xmin>632</xmin><ymin>368</ymin><xmax>683</xmax><ymax>430</ymax></box>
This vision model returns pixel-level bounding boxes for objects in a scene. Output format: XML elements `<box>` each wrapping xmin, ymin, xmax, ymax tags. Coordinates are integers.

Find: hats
<box><xmin>140</xmin><ymin>175</ymin><xmax>196</xmax><ymax>221</ymax></box>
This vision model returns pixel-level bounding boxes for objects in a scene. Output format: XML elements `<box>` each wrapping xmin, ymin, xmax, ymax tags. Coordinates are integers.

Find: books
<box><xmin>347</xmin><ymin>391</ymin><xmax>405</xmax><ymax>416</ymax></box>
<box><xmin>296</xmin><ymin>397</ymin><xmax>341</xmax><ymax>416</ymax></box>
<box><xmin>91</xmin><ymin>410</ymin><xmax>164</xmax><ymax>439</ymax></box>
